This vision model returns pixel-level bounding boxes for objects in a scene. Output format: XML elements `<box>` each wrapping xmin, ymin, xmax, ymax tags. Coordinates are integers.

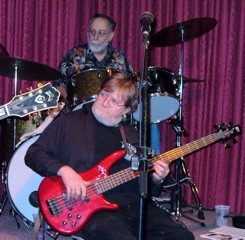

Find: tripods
<box><xmin>161</xmin><ymin>30</ymin><xmax>206</xmax><ymax>218</ymax></box>
<box><xmin>0</xmin><ymin>65</ymin><xmax>22</xmax><ymax>228</ymax></box>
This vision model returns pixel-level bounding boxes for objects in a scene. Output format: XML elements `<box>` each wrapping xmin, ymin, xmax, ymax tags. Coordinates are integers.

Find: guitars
<box><xmin>0</xmin><ymin>82</ymin><xmax>61</xmax><ymax>120</ymax></box>
<box><xmin>37</xmin><ymin>121</ymin><xmax>241</xmax><ymax>234</ymax></box>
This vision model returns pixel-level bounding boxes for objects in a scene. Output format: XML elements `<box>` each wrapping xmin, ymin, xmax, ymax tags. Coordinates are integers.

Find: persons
<box><xmin>54</xmin><ymin>14</ymin><xmax>135</xmax><ymax>104</ymax></box>
<box><xmin>27</xmin><ymin>75</ymin><xmax>195</xmax><ymax>240</ymax></box>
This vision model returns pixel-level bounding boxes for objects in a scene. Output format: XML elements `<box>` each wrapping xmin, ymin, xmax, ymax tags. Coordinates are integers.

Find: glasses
<box><xmin>97</xmin><ymin>91</ymin><xmax>128</xmax><ymax>107</ymax></box>
<box><xmin>87</xmin><ymin>29</ymin><xmax>113</xmax><ymax>36</ymax></box>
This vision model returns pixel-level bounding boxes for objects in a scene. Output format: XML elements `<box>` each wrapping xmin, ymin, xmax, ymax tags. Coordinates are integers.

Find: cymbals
<box><xmin>149</xmin><ymin>16</ymin><xmax>217</xmax><ymax>48</ymax></box>
<box><xmin>175</xmin><ymin>73</ymin><xmax>205</xmax><ymax>82</ymax></box>
<box><xmin>0</xmin><ymin>56</ymin><xmax>62</xmax><ymax>82</ymax></box>
<box><xmin>0</xmin><ymin>44</ymin><xmax>9</xmax><ymax>56</ymax></box>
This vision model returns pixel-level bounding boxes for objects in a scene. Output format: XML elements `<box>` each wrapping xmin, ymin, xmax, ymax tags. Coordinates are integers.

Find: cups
<box><xmin>215</xmin><ymin>205</ymin><xmax>230</xmax><ymax>226</ymax></box>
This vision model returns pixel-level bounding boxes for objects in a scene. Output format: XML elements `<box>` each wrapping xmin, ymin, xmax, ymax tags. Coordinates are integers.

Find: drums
<box><xmin>66</xmin><ymin>68</ymin><xmax>113</xmax><ymax>111</ymax></box>
<box><xmin>7</xmin><ymin>112</ymin><xmax>42</xmax><ymax>136</ymax></box>
<box><xmin>131</xmin><ymin>66</ymin><xmax>181</xmax><ymax>123</ymax></box>
<box><xmin>2</xmin><ymin>133</ymin><xmax>47</xmax><ymax>227</ymax></box>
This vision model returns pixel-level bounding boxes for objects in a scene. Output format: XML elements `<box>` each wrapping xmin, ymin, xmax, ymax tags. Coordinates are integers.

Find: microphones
<box><xmin>139</xmin><ymin>11</ymin><xmax>154</xmax><ymax>35</ymax></box>
